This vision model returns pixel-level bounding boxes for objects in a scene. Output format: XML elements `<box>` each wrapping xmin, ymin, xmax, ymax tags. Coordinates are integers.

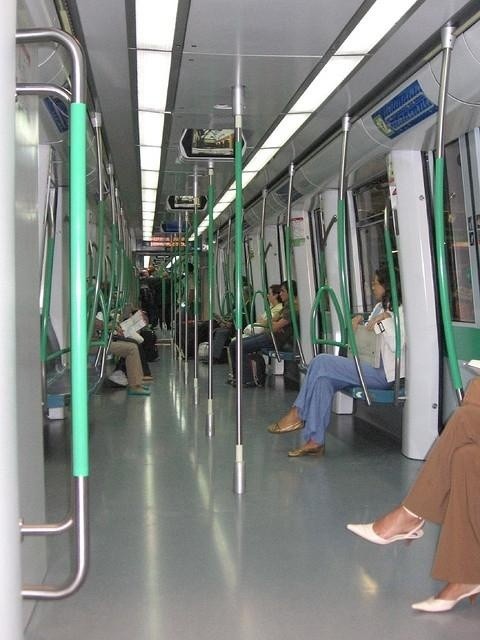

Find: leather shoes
<box><xmin>287</xmin><ymin>444</ymin><xmax>326</xmax><ymax>458</ymax></box>
<box><xmin>266</xmin><ymin>418</ymin><xmax>305</xmax><ymax>433</ymax></box>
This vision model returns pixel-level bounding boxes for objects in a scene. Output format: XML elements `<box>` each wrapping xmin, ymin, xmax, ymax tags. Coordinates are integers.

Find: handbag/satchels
<box><xmin>243</xmin><ymin>322</ymin><xmax>265</xmax><ymax>336</ymax></box>
<box><xmin>345</xmin><ymin>319</ymin><xmax>382</xmax><ymax>370</ymax></box>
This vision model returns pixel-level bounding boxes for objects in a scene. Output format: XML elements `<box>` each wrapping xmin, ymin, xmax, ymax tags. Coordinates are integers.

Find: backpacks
<box><xmin>246</xmin><ymin>354</ymin><xmax>268</xmax><ymax>386</ymax></box>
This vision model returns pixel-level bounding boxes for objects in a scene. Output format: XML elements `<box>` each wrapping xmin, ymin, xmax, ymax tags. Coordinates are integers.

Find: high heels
<box><xmin>410</xmin><ymin>581</ymin><xmax>480</xmax><ymax>615</ymax></box>
<box><xmin>344</xmin><ymin>509</ymin><xmax>426</xmax><ymax>546</ymax></box>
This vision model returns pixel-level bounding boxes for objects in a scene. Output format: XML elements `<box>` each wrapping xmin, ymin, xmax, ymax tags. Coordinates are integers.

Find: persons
<box><xmin>265</xmin><ymin>262</ymin><xmax>407</xmax><ymax>458</ymax></box>
<box><xmin>229</xmin><ymin>279</ymin><xmax>299</xmax><ymax>388</ymax></box>
<box><xmin>342</xmin><ymin>377</ymin><xmax>480</xmax><ymax>615</ymax></box>
<box><xmin>225</xmin><ymin>284</ymin><xmax>285</xmax><ymax>388</ymax></box>
<box><xmin>81</xmin><ymin>261</ymin><xmax>195</xmax><ymax>398</ymax></box>
<box><xmin>200</xmin><ymin>286</ymin><xmax>256</xmax><ymax>365</ymax></box>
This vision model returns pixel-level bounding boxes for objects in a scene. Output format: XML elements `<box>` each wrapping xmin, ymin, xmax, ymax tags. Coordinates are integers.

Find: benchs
<box><xmin>38</xmin><ymin>306</ymin><xmax>120</xmax><ymax>421</ymax></box>
<box><xmin>218</xmin><ymin>288</ymin><xmax>404</xmax><ymax>407</ymax></box>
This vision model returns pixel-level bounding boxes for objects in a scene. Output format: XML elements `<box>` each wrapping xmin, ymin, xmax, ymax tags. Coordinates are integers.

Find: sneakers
<box><xmin>107</xmin><ymin>371</ymin><xmax>155</xmax><ymax>396</ymax></box>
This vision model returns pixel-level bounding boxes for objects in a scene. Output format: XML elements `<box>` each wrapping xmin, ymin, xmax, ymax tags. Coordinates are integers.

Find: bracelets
<box><xmin>383</xmin><ymin>312</ymin><xmax>387</xmax><ymax>320</ymax></box>
